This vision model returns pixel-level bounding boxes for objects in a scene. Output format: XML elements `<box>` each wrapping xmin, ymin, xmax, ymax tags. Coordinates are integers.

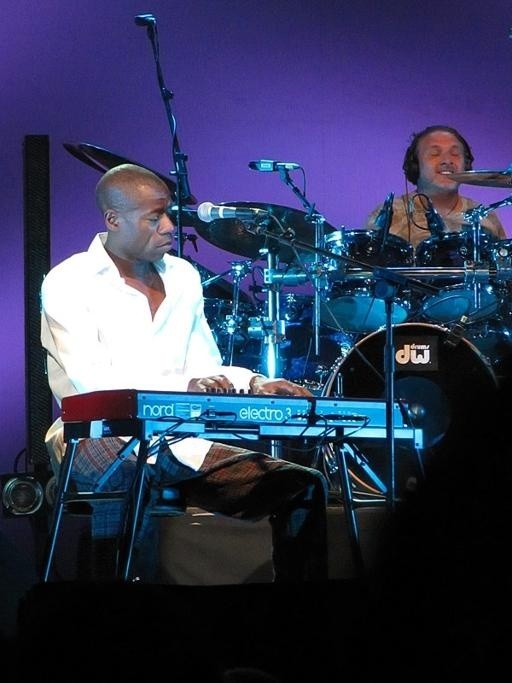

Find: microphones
<box><xmin>197</xmin><ymin>201</ymin><xmax>272</xmax><ymax>223</ymax></box>
<box><xmin>250</xmin><ymin>160</ymin><xmax>302</xmax><ymax>171</ymax></box>
<box><xmin>377</xmin><ymin>194</ymin><xmax>394</xmax><ymax>229</ymax></box>
<box><xmin>410</xmin><ymin>194</ymin><xmax>446</xmax><ymax>234</ymax></box>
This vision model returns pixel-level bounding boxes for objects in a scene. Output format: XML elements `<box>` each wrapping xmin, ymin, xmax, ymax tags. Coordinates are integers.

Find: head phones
<box><xmin>404</xmin><ymin>125</ymin><xmax>474</xmax><ymax>183</ymax></box>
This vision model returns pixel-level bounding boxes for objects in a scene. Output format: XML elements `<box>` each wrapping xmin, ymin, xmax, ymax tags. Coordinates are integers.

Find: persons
<box><xmin>370</xmin><ymin>125</ymin><xmax>507</xmax><ymax>248</ymax></box>
<box><xmin>41</xmin><ymin>163</ymin><xmax>334</xmax><ymax>582</ymax></box>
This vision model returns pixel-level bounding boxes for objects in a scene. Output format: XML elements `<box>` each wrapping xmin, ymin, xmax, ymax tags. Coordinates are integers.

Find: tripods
<box><xmin>308</xmin><ymin>375</ymin><xmax>389</xmax><ymax>504</ymax></box>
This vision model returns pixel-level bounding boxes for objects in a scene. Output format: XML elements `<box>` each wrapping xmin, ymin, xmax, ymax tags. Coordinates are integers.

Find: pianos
<box><xmin>61</xmin><ymin>388</ymin><xmax>414</xmax><ymax>426</ymax></box>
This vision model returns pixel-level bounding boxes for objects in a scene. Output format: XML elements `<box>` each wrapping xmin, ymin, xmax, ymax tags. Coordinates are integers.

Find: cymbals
<box><xmin>193</xmin><ymin>200</ymin><xmax>339</xmax><ymax>263</ymax></box>
<box><xmin>446</xmin><ymin>169</ymin><xmax>512</xmax><ymax>189</ymax></box>
<box><xmin>62</xmin><ymin>138</ymin><xmax>197</xmax><ymax>205</ymax></box>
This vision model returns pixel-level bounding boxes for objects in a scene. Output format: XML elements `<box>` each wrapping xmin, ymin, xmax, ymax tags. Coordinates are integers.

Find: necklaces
<box><xmin>417</xmin><ymin>189</ymin><xmax>459</xmax><ymax>219</ymax></box>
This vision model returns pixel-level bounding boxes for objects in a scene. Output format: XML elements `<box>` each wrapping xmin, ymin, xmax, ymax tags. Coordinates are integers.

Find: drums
<box><xmin>256</xmin><ymin>291</ymin><xmax>342</xmax><ymax>388</ymax></box>
<box><xmin>315</xmin><ymin>227</ymin><xmax>415</xmax><ymax>334</ymax></box>
<box><xmin>415</xmin><ymin>230</ymin><xmax>501</xmax><ymax>327</ymax></box>
<box><xmin>324</xmin><ymin>322</ymin><xmax>500</xmax><ymax>503</ymax></box>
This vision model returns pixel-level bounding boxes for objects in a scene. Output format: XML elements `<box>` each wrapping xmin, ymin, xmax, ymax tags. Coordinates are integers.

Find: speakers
<box><xmin>22</xmin><ymin>132</ymin><xmax>52</xmax><ymax>469</ymax></box>
<box><xmin>0</xmin><ymin>472</ymin><xmax>80</xmax><ymax>516</ymax></box>
